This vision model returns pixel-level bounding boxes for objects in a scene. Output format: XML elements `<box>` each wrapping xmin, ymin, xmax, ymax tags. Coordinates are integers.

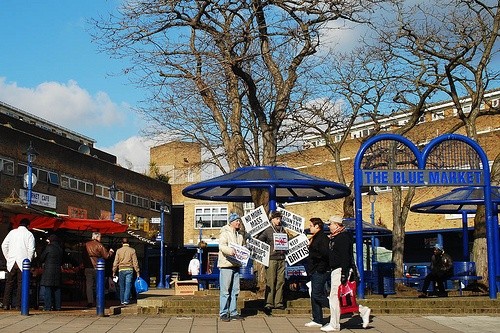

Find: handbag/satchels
<box><xmin>134</xmin><ymin>277</ymin><xmax>148</xmax><ymax>295</ymax></box>
<box><xmin>338</xmin><ymin>281</ymin><xmax>359</xmax><ymax>314</ymax></box>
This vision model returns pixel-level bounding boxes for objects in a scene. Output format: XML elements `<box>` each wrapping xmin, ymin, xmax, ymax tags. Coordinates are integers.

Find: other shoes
<box><xmin>277</xmin><ymin>306</ymin><xmax>285</xmax><ymax>310</ymax></box>
<box><xmin>360</xmin><ymin>306</ymin><xmax>371</xmax><ymax>328</ymax></box>
<box><xmin>121</xmin><ymin>301</ymin><xmax>129</xmax><ymax>305</ymax></box>
<box><xmin>15</xmin><ymin>306</ymin><xmax>20</xmax><ymax>311</ymax></box>
<box><xmin>262</xmin><ymin>306</ymin><xmax>271</xmax><ymax>316</ymax></box>
<box><xmin>305</xmin><ymin>321</ymin><xmax>322</xmax><ymax>327</ymax></box>
<box><xmin>4</xmin><ymin>306</ymin><xmax>10</xmax><ymax>310</ymax></box>
<box><xmin>221</xmin><ymin>314</ymin><xmax>230</xmax><ymax>322</ymax></box>
<box><xmin>231</xmin><ymin>316</ymin><xmax>237</xmax><ymax>320</ymax></box>
<box><xmin>438</xmin><ymin>293</ymin><xmax>448</xmax><ymax>297</ymax></box>
<box><xmin>0</xmin><ymin>302</ymin><xmax>4</xmax><ymax>308</ymax></box>
<box><xmin>320</xmin><ymin>323</ymin><xmax>340</xmax><ymax>331</ymax></box>
<box><xmin>419</xmin><ymin>294</ymin><xmax>427</xmax><ymax>298</ymax></box>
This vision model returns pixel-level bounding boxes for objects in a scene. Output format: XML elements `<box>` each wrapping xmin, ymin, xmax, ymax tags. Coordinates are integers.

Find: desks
<box><xmin>15</xmin><ymin>271</ymin><xmax>83</xmax><ymax>308</ymax></box>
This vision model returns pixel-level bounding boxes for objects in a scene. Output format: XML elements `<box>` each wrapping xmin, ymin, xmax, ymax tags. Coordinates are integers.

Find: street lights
<box><xmin>365</xmin><ymin>186</ymin><xmax>379</xmax><ymax>266</ymax></box>
<box><xmin>108</xmin><ymin>179</ymin><xmax>121</xmax><ymax>252</ymax></box>
<box><xmin>21</xmin><ymin>140</ymin><xmax>38</xmax><ymax>206</ymax></box>
<box><xmin>196</xmin><ymin>215</ymin><xmax>206</xmax><ymax>273</ymax></box>
<box><xmin>156</xmin><ymin>198</ymin><xmax>172</xmax><ymax>289</ymax></box>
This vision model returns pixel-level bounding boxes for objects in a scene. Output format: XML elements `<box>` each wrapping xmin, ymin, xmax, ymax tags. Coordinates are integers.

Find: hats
<box><xmin>434</xmin><ymin>244</ymin><xmax>444</xmax><ymax>251</ymax></box>
<box><xmin>229</xmin><ymin>213</ymin><xmax>241</xmax><ymax>223</ymax></box>
<box><xmin>269</xmin><ymin>211</ymin><xmax>283</xmax><ymax>221</ymax></box>
<box><xmin>329</xmin><ymin>217</ymin><xmax>343</xmax><ymax>224</ymax></box>
<box><xmin>18</xmin><ymin>218</ymin><xmax>29</xmax><ymax>227</ymax></box>
<box><xmin>48</xmin><ymin>235</ymin><xmax>57</xmax><ymax>241</ymax></box>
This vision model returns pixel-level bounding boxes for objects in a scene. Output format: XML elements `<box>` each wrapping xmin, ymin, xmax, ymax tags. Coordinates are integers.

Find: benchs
<box><xmin>395</xmin><ymin>261</ymin><xmax>482</xmax><ymax>297</ymax></box>
<box><xmin>191</xmin><ymin>258</ymin><xmax>254</xmax><ymax>290</ymax></box>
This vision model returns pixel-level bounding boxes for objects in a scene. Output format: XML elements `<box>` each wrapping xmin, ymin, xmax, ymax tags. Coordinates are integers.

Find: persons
<box><xmin>82</xmin><ymin>230</ymin><xmax>114</xmax><ymax>309</ymax></box>
<box><xmin>188</xmin><ymin>253</ymin><xmax>201</xmax><ymax>283</ymax></box>
<box><xmin>254</xmin><ymin>211</ymin><xmax>294</xmax><ymax>311</ymax></box>
<box><xmin>304</xmin><ymin>218</ymin><xmax>333</xmax><ymax>328</ymax></box>
<box><xmin>321</xmin><ymin>216</ymin><xmax>372</xmax><ymax>332</ymax></box>
<box><xmin>111</xmin><ymin>237</ymin><xmax>141</xmax><ymax>305</ymax></box>
<box><xmin>0</xmin><ymin>214</ymin><xmax>65</xmax><ymax>313</ymax></box>
<box><xmin>216</xmin><ymin>212</ymin><xmax>248</xmax><ymax>322</ymax></box>
<box><xmin>418</xmin><ymin>244</ymin><xmax>453</xmax><ymax>300</ymax></box>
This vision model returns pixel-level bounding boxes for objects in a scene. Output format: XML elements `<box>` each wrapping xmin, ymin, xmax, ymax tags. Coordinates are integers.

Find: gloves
<box><xmin>341</xmin><ymin>275</ymin><xmax>348</xmax><ymax>285</ymax></box>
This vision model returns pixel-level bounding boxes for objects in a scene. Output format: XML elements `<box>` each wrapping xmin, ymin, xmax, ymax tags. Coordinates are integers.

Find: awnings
<box><xmin>0</xmin><ymin>203</ymin><xmax>156</xmax><ymax>244</ymax></box>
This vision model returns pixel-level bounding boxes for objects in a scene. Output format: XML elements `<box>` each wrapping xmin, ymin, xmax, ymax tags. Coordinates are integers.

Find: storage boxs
<box><xmin>169</xmin><ymin>276</ymin><xmax>198</xmax><ymax>295</ymax></box>
